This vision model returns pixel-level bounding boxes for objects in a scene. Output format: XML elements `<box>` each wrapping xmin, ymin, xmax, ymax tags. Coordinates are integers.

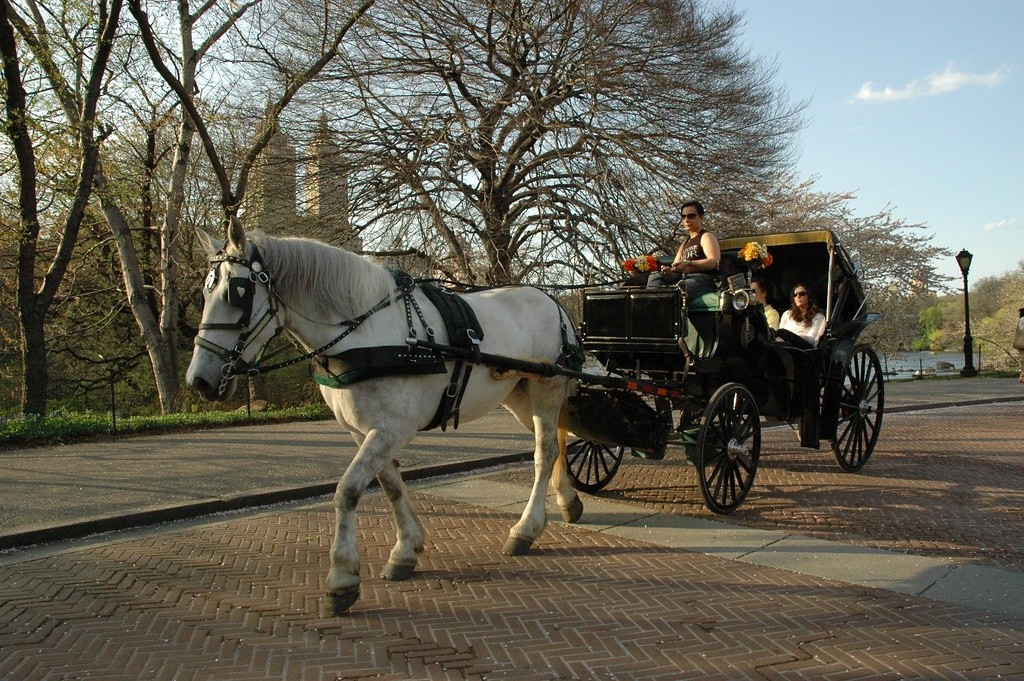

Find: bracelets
<box><xmin>683</xmin><ymin>259</ymin><xmax>689</xmax><ymax>264</ymax></box>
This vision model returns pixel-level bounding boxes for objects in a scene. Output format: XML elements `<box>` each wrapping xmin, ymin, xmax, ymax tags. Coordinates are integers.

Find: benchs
<box><xmin>623</xmin><ymin>251</ymin><xmax>751</xmax><ymax>311</ymax></box>
<box><xmin>776</xmin><ymin>306</ymin><xmax>833</xmax><ymax>386</ymax></box>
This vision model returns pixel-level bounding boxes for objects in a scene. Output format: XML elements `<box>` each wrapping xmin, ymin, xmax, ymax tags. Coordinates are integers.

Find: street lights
<box><xmin>955</xmin><ymin>247</ymin><xmax>979</xmax><ymax>378</ymax></box>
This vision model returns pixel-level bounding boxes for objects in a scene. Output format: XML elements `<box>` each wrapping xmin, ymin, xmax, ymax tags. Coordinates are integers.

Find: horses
<box><xmin>183</xmin><ymin>214</ymin><xmax>584</xmax><ymax>620</ymax></box>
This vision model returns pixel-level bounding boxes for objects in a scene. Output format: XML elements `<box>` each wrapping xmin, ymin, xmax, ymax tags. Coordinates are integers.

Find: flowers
<box><xmin>623</xmin><ymin>255</ymin><xmax>658</xmax><ymax>286</ymax></box>
<box><xmin>738</xmin><ymin>241</ymin><xmax>773</xmax><ymax>273</ymax></box>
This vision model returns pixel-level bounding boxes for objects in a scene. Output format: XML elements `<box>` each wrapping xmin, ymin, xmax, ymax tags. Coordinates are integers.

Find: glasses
<box><xmin>792</xmin><ymin>290</ymin><xmax>808</xmax><ymax>297</ymax></box>
<box><xmin>751</xmin><ymin>288</ymin><xmax>764</xmax><ymax>293</ymax></box>
<box><xmin>680</xmin><ymin>213</ymin><xmax>700</xmax><ymax>219</ymax></box>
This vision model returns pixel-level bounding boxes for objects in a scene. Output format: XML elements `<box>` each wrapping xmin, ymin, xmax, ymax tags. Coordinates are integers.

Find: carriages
<box><xmin>186</xmin><ymin>214</ymin><xmax>885</xmax><ymax>618</ymax></box>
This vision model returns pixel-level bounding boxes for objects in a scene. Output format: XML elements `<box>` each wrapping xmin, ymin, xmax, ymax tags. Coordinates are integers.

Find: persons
<box><xmin>660</xmin><ymin>200</ymin><xmax>825</xmax><ymax>351</ymax></box>
<box><xmin>1013</xmin><ymin>307</ymin><xmax>1024</xmax><ymax>382</ymax></box>
